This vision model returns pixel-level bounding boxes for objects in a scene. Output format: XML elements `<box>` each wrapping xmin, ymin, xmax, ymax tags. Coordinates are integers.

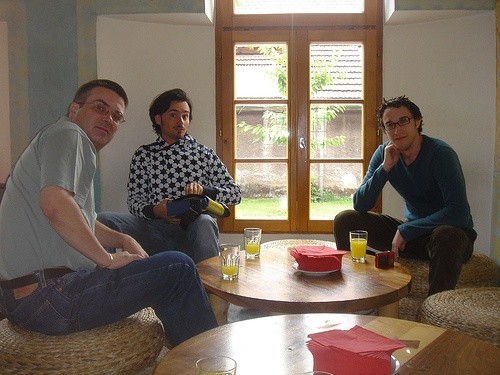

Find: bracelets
<box><xmin>97</xmin><ymin>253</ymin><xmax>114</xmax><ymax>270</ymax></box>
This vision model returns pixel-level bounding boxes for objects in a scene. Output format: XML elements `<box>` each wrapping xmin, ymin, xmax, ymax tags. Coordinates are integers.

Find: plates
<box><xmin>292</xmin><ymin>261</ymin><xmax>339</xmax><ymax>276</ymax></box>
<box><xmin>388</xmin><ymin>355</ymin><xmax>401</xmax><ymax>375</ymax></box>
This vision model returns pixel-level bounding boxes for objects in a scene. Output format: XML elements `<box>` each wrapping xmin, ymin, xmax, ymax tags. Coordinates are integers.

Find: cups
<box><xmin>300</xmin><ymin>371</ymin><xmax>335</xmax><ymax>375</ymax></box>
<box><xmin>195</xmin><ymin>355</ymin><xmax>237</xmax><ymax>375</ymax></box>
<box><xmin>219</xmin><ymin>243</ymin><xmax>240</xmax><ymax>280</ymax></box>
<box><xmin>349</xmin><ymin>230</ymin><xmax>368</xmax><ymax>264</ymax></box>
<box><xmin>244</xmin><ymin>227</ymin><xmax>263</xmax><ymax>260</ymax></box>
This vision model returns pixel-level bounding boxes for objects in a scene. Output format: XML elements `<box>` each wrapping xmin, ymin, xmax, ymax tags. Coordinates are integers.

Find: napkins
<box><xmin>306</xmin><ymin>325</ymin><xmax>408</xmax><ymax>375</ymax></box>
<box><xmin>290</xmin><ymin>246</ymin><xmax>348</xmax><ymax>271</ymax></box>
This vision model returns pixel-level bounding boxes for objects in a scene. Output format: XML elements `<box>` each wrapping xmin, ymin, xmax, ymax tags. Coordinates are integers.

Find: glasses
<box><xmin>384</xmin><ymin>115</ymin><xmax>412</xmax><ymax>130</ymax></box>
<box><xmin>75</xmin><ymin>100</ymin><xmax>127</xmax><ymax>124</ymax></box>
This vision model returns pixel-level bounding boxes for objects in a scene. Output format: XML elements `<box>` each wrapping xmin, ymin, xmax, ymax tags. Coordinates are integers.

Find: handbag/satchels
<box><xmin>166</xmin><ymin>192</ymin><xmax>229</xmax><ymax>232</ymax></box>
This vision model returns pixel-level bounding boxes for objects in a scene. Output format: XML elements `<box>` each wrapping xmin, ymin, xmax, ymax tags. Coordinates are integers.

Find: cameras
<box><xmin>375</xmin><ymin>251</ymin><xmax>395</xmax><ymax>269</ymax></box>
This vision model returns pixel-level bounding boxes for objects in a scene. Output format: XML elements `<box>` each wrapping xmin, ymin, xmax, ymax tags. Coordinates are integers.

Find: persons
<box><xmin>96</xmin><ymin>88</ymin><xmax>241</xmax><ymax>264</ymax></box>
<box><xmin>0</xmin><ymin>79</ymin><xmax>218</xmax><ymax>347</ymax></box>
<box><xmin>333</xmin><ymin>95</ymin><xmax>478</xmax><ymax>298</ymax></box>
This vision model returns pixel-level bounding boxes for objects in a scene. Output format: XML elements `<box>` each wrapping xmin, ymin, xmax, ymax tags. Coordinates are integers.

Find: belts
<box><xmin>0</xmin><ymin>268</ymin><xmax>77</xmax><ymax>290</ymax></box>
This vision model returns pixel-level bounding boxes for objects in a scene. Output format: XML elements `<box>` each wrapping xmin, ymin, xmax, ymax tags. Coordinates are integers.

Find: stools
<box><xmin>0</xmin><ymin>307</ymin><xmax>166</xmax><ymax>375</ymax></box>
<box><xmin>415</xmin><ymin>287</ymin><xmax>500</xmax><ymax>348</ymax></box>
<box><xmin>397</xmin><ymin>251</ymin><xmax>500</xmax><ymax>323</ymax></box>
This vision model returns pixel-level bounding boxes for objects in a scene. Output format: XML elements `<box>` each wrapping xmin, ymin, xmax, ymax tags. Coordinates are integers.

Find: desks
<box><xmin>153</xmin><ymin>312</ymin><xmax>500</xmax><ymax>375</ymax></box>
<box><xmin>196</xmin><ymin>238</ymin><xmax>412</xmax><ymax>320</ymax></box>
